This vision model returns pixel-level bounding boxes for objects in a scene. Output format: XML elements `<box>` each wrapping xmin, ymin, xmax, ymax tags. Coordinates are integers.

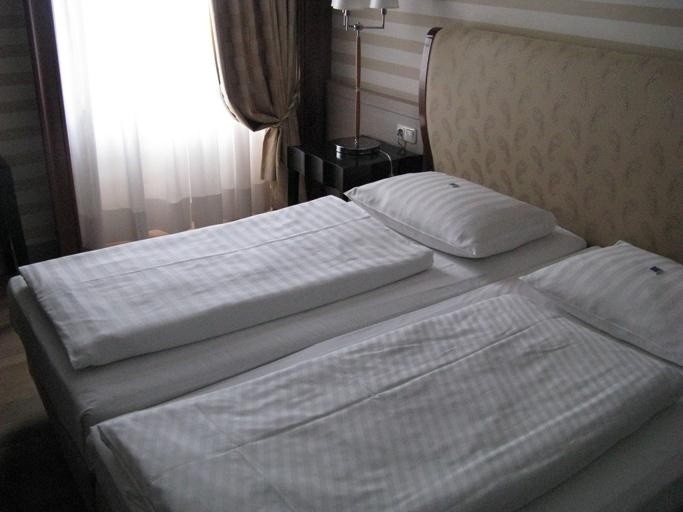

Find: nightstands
<box><xmin>284</xmin><ymin>133</ymin><xmax>423</xmax><ymax>205</ymax></box>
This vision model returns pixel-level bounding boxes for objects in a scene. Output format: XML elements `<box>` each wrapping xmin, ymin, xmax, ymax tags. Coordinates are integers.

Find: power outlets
<box><xmin>396</xmin><ymin>123</ymin><xmax>405</xmax><ymax>141</ymax></box>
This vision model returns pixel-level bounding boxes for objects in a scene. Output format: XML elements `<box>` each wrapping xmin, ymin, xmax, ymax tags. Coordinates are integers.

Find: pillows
<box><xmin>341</xmin><ymin>170</ymin><xmax>558</xmax><ymax>259</ymax></box>
<box><xmin>517</xmin><ymin>239</ymin><xmax>682</xmax><ymax>370</ymax></box>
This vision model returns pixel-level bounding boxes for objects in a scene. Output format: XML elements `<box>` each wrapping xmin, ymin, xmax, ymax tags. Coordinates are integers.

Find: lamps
<box><xmin>329</xmin><ymin>0</ymin><xmax>399</xmax><ymax>153</ymax></box>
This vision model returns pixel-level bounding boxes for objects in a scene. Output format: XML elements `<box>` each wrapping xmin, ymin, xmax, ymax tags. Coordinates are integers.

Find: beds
<box><xmin>5</xmin><ymin>15</ymin><xmax>682</xmax><ymax>512</ymax></box>
